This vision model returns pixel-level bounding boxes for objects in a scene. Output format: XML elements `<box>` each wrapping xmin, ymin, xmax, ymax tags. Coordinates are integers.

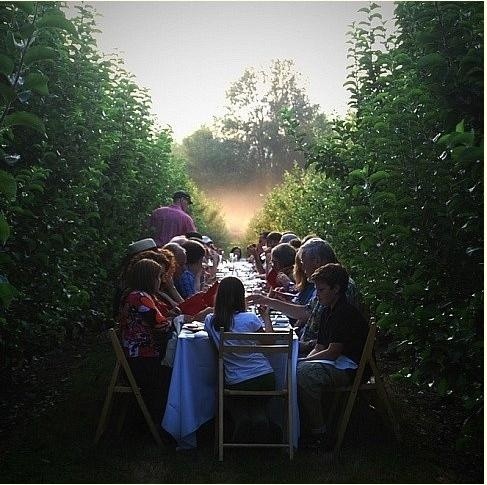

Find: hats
<box><xmin>173</xmin><ymin>191</ymin><xmax>192</xmax><ymax>204</ymax></box>
<box><xmin>127</xmin><ymin>238</ymin><xmax>156</xmax><ymax>256</ymax></box>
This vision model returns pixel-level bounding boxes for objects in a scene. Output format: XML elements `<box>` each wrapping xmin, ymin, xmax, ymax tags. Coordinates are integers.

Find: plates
<box><xmin>181</xmin><ymin>325</ymin><xmax>205</xmax><ymax>331</ymax></box>
<box><xmin>249</xmin><ymin>302</ymin><xmax>289</xmax><ymax>328</ymax></box>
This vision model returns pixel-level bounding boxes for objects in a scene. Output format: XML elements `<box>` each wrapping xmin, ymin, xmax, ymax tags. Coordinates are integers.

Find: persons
<box><xmin>115</xmin><ymin>191</ymin><xmax>376</xmax><ymax>442</ymax></box>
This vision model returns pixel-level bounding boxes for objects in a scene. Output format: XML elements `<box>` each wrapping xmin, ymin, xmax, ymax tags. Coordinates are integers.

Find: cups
<box><xmin>204</xmin><ymin>250</ymin><xmax>267</xmax><ymax>292</ymax></box>
